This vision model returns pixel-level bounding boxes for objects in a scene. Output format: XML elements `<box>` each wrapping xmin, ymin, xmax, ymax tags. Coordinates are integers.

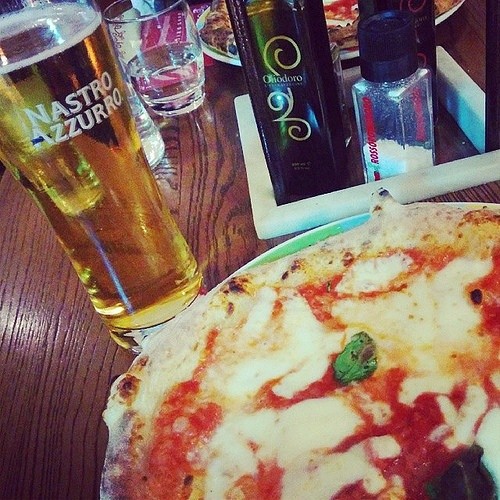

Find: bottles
<box><xmin>352</xmin><ymin>9</ymin><xmax>437</xmax><ymax>185</ymax></box>
<box><xmin>224</xmin><ymin>0</ymin><xmax>353</xmax><ymax>205</ymax></box>
<box><xmin>356</xmin><ymin>0</ymin><xmax>435</xmax><ymax>127</ymax></box>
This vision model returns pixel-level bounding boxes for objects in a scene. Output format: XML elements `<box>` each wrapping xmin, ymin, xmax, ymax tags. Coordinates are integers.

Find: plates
<box><xmin>196</xmin><ymin>0</ymin><xmax>465</xmax><ymax>66</ymax></box>
<box><xmin>100</xmin><ymin>203</ymin><xmax>500</xmax><ymax>500</ymax></box>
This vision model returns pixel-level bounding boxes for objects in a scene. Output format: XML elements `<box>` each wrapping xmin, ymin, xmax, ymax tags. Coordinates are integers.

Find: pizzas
<box><xmin>197</xmin><ymin>0</ymin><xmax>463</xmax><ymax>57</ymax></box>
<box><xmin>102</xmin><ymin>186</ymin><xmax>500</xmax><ymax>500</ymax></box>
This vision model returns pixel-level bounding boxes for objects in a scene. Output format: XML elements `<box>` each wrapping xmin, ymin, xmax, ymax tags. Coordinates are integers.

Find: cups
<box><xmin>0</xmin><ymin>0</ymin><xmax>203</xmax><ymax>351</ymax></box>
<box><xmin>101</xmin><ymin>0</ymin><xmax>204</xmax><ymax>116</ymax></box>
<box><xmin>19</xmin><ymin>0</ymin><xmax>166</xmax><ymax>175</ymax></box>
